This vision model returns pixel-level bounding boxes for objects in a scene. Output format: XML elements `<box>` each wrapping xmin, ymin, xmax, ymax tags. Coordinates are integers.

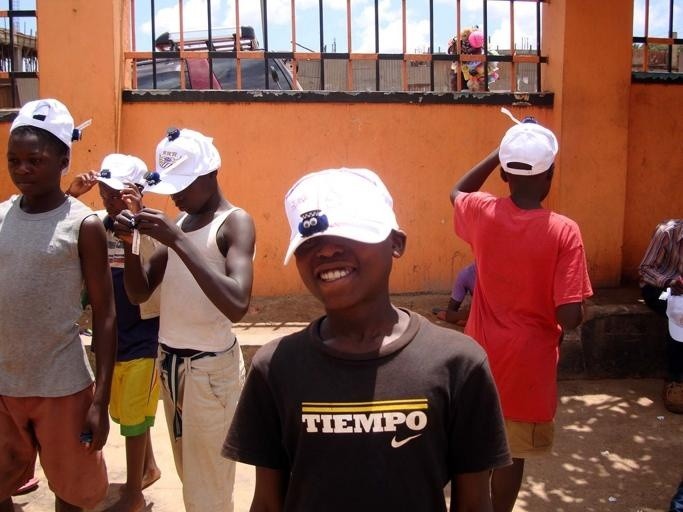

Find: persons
<box><xmin>635</xmin><ymin>209</ymin><xmax>682</xmax><ymax>383</ymax></box>
<box><xmin>446</xmin><ymin>118</ymin><xmax>598</xmax><ymax>512</ymax></box>
<box><xmin>0</xmin><ymin>94</ymin><xmax>118</xmax><ymax>512</ymax></box>
<box><xmin>217</xmin><ymin>162</ymin><xmax>515</xmax><ymax>512</ymax></box>
<box><xmin>427</xmin><ymin>256</ymin><xmax>479</xmax><ymax>329</ymax></box>
<box><xmin>61</xmin><ymin>151</ymin><xmax>161</xmax><ymax>512</ymax></box>
<box><xmin>110</xmin><ymin>122</ymin><xmax>259</xmax><ymax>512</ymax></box>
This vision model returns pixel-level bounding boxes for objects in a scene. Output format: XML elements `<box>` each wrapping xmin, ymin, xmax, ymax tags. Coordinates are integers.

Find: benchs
<box><xmin>578</xmin><ymin>300</ymin><xmax>671</xmax><ymax>377</ymax></box>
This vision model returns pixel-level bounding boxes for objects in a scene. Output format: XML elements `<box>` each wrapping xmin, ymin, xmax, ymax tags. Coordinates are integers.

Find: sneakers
<box><xmin>663</xmin><ymin>382</ymin><xmax>683</xmax><ymax>413</ymax></box>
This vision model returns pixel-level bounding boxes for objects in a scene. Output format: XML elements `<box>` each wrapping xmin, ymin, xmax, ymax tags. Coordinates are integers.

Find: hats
<box><xmin>666</xmin><ymin>287</ymin><xmax>683</xmax><ymax>342</ymax></box>
<box><xmin>143</xmin><ymin>130</ymin><xmax>222</xmax><ymax>196</ymax></box>
<box><xmin>283</xmin><ymin>168</ymin><xmax>400</xmax><ymax>265</ymax></box>
<box><xmin>10</xmin><ymin>99</ymin><xmax>74</xmax><ymax>175</ymax></box>
<box><xmin>91</xmin><ymin>152</ymin><xmax>148</xmax><ymax>191</ymax></box>
<box><xmin>498</xmin><ymin>123</ymin><xmax>557</xmax><ymax>176</ymax></box>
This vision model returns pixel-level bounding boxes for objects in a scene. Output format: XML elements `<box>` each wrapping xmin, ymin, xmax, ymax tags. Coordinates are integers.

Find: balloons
<box><xmin>468</xmin><ymin>31</ymin><xmax>485</xmax><ymax>49</ymax></box>
